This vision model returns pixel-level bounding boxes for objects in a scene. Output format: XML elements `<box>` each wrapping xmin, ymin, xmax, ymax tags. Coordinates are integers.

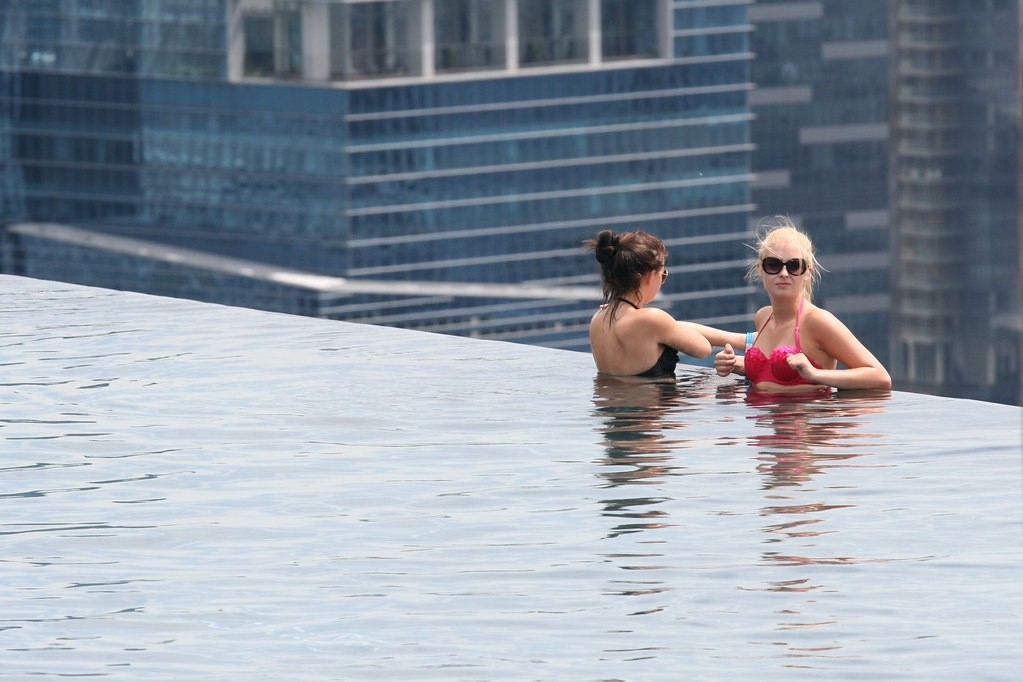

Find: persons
<box><xmin>714</xmin><ymin>221</ymin><xmax>892</xmax><ymax>396</ymax></box>
<box><xmin>584</xmin><ymin>229</ymin><xmax>757</xmax><ymax>390</ymax></box>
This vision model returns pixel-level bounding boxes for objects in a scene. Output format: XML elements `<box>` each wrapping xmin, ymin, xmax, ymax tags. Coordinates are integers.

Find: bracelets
<box><xmin>745</xmin><ymin>332</ymin><xmax>754</xmax><ymax>354</ymax></box>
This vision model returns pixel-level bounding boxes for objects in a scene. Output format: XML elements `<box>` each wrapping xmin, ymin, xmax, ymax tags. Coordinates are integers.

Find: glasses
<box><xmin>656</xmin><ymin>270</ymin><xmax>668</xmax><ymax>284</ymax></box>
<box><xmin>763</xmin><ymin>256</ymin><xmax>807</xmax><ymax>276</ymax></box>
